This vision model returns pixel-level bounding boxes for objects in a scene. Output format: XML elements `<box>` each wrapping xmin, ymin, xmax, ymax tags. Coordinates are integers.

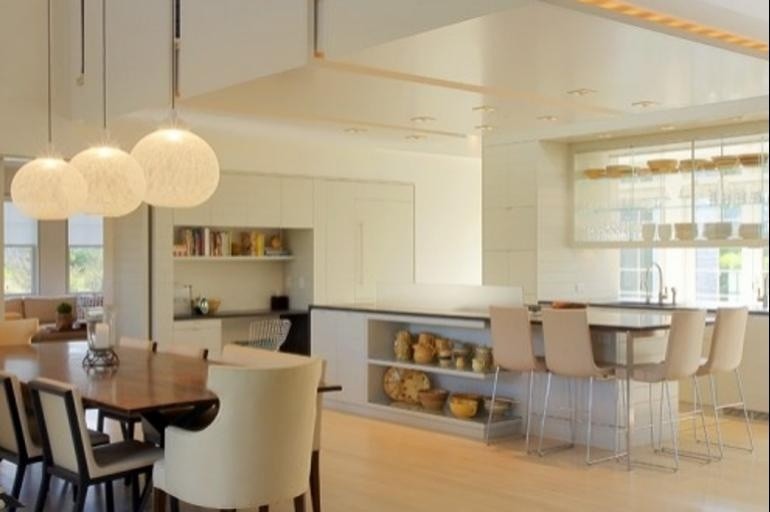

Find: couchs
<box><xmin>4</xmin><ymin>296</ymin><xmax>87</xmax><ymax>341</ymax></box>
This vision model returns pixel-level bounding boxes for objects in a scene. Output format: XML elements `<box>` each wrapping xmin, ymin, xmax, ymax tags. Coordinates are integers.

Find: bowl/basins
<box><xmin>417</xmin><ymin>387</ymin><xmax>515</xmax><ymax>418</ymax></box>
<box><xmin>583</xmin><ymin>153</ymin><xmax>769</xmax><ymax>180</ymax></box>
<box><xmin>640</xmin><ymin>221</ymin><xmax>762</xmax><ymax>240</ymax></box>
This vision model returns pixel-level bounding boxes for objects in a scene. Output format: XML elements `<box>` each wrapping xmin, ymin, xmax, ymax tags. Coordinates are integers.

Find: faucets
<box><xmin>645</xmin><ymin>262</ymin><xmax>668</xmax><ymax>305</ymax></box>
<box><xmin>757</xmin><ymin>275</ymin><xmax>768</xmax><ymax>306</ymax></box>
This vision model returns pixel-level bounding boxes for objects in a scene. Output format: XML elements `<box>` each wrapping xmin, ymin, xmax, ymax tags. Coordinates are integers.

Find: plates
<box><xmin>382</xmin><ymin>366</ymin><xmax>432</xmax><ymax>405</ymax></box>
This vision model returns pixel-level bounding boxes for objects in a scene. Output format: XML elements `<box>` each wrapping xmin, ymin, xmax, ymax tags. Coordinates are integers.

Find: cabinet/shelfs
<box><xmin>252</xmin><ymin>176</ymin><xmax>314</xmax><ymax>228</ymax></box>
<box><xmin>312</xmin><ymin>308</ymin><xmax>368</xmax><ymax>401</ymax></box>
<box><xmin>173</xmin><ymin>173</ymin><xmax>251</xmax><ymax>223</ymax></box>
<box><xmin>174</xmin><ymin>226</ymin><xmax>294</xmax><ymax>261</ymax></box>
<box><xmin>323</xmin><ymin>192</ymin><xmax>412</xmax><ymax>303</ymax></box>
<box><xmin>174</xmin><ymin>322</ymin><xmax>221</xmax><ymax>357</ymax></box>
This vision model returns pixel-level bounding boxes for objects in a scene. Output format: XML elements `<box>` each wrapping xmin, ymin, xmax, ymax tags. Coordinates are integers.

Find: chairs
<box><xmin>538</xmin><ymin>309</ymin><xmax>626</xmax><ymax>464</ymax></box>
<box><xmin>248</xmin><ymin>319</ymin><xmax>290</xmax><ymax>350</ymax></box>
<box><xmin>1</xmin><ymin>373</ymin><xmax>113</xmax><ymax>509</ymax></box>
<box><xmin>661</xmin><ymin>306</ymin><xmax>755</xmax><ymax>458</ymax></box>
<box><xmin>151</xmin><ymin>359</ymin><xmax>321</xmax><ymax>510</ymax></box>
<box><xmin>1</xmin><ymin>318</ymin><xmax>39</xmax><ymax>347</ymax></box>
<box><xmin>124</xmin><ymin>340</ymin><xmax>205</xmax><ymax>487</ymax></box>
<box><xmin>221</xmin><ymin>344</ymin><xmax>320</xmax><ymax>508</ymax></box>
<box><xmin>614</xmin><ymin>306</ymin><xmax>709</xmax><ymax>471</ymax></box>
<box><xmin>484</xmin><ymin>303</ymin><xmax>574</xmax><ymax>452</ymax></box>
<box><xmin>26</xmin><ymin>380</ymin><xmax>166</xmax><ymax>510</ymax></box>
<box><xmin>99</xmin><ymin>341</ymin><xmax>153</xmax><ymax>447</ymax></box>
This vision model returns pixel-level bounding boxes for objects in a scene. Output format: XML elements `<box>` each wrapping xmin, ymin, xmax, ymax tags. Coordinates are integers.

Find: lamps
<box><xmin>10</xmin><ymin>1</ymin><xmax>221</xmax><ymax>222</ymax></box>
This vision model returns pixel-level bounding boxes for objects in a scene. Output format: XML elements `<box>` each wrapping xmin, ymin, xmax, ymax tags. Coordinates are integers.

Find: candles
<box><xmin>95</xmin><ymin>323</ymin><xmax>109</xmax><ymax>348</ymax></box>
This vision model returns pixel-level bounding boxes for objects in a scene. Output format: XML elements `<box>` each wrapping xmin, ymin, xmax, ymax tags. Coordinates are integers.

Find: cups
<box><xmin>471</xmin><ymin>358</ymin><xmax>485</xmax><ymax>372</ymax></box>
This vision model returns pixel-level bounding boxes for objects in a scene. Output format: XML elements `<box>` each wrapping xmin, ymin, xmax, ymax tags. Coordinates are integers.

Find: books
<box><xmin>172</xmin><ymin>227</ymin><xmax>290</xmax><ymax>255</ymax></box>
